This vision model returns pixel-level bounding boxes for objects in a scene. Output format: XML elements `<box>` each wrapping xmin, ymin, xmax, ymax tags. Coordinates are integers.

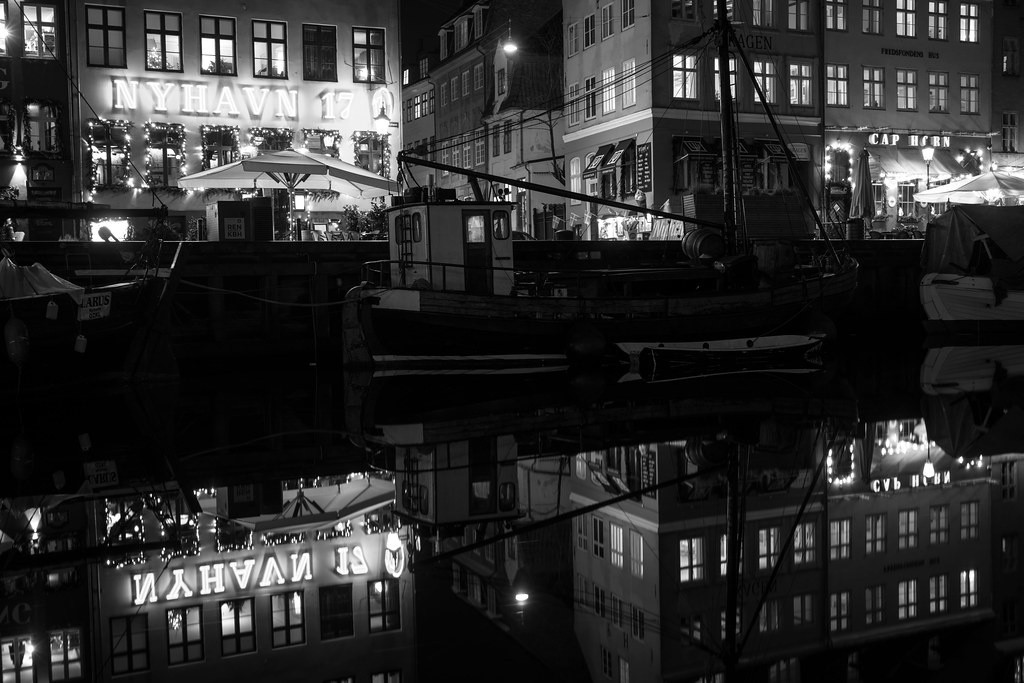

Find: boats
<box><xmin>914</xmin><ymin>333</ymin><xmax>1024</xmax><ymax>410</ymax></box>
<box><xmin>917</xmin><ymin>274</ymin><xmax>1024</xmax><ymax>336</ymax></box>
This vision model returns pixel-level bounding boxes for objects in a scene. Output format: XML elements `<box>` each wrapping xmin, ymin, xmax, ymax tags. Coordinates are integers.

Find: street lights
<box><xmin>514</xmin><ymin>533</ymin><xmax>531</xmax><ymax>604</ymax></box>
<box><xmin>920</xmin><ymin>411</ymin><xmax>936</xmax><ymax>479</ymax></box>
<box><xmin>476</xmin><ymin>6</ymin><xmax>519</xmax><ymax>198</ymax></box>
<box><xmin>922</xmin><ymin>145</ymin><xmax>935</xmax><ymax>225</ymax></box>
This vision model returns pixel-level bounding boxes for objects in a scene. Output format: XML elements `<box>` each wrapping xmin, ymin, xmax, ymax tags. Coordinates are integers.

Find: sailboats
<box><xmin>0</xmin><ymin>408</ymin><xmax>184</xmax><ymax>681</ymax></box>
<box><xmin>0</xmin><ymin>3</ymin><xmax>182</xmax><ymax>412</ymax></box>
<box><xmin>353</xmin><ymin>0</ymin><xmax>865</xmax><ymax>385</ymax></box>
<box><xmin>346</xmin><ymin>379</ymin><xmax>866</xmax><ymax>683</ymax></box>
